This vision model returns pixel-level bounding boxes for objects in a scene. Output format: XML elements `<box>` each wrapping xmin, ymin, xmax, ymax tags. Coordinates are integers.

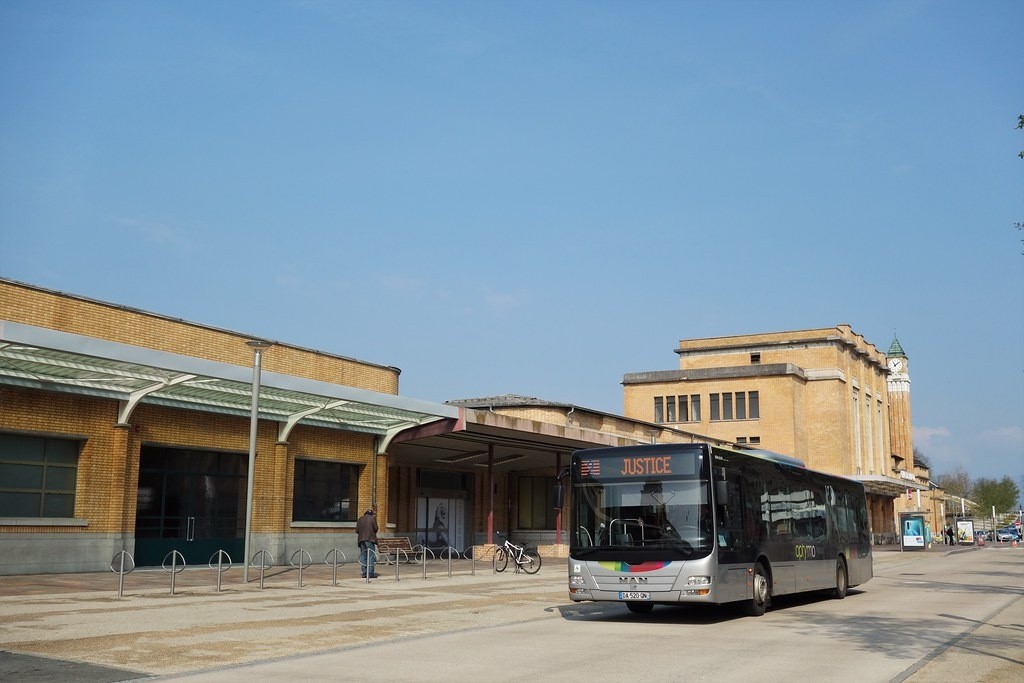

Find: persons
<box><xmin>694</xmin><ymin>505</ymin><xmax>708</xmax><ymax>531</ymax></box>
<box><xmin>356</xmin><ymin>509</ymin><xmax>378</xmax><ymax>578</ymax></box>
<box><xmin>941</xmin><ymin>528</ymin><xmax>947</xmax><ymax>544</ymax></box>
<box><xmin>947</xmin><ymin>526</ymin><xmax>954</xmax><ymax>546</ymax></box>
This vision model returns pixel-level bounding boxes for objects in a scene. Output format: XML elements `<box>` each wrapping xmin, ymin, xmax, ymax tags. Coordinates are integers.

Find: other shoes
<box><xmin>363</xmin><ymin>573</ymin><xmax>378</xmax><ymax>579</ymax></box>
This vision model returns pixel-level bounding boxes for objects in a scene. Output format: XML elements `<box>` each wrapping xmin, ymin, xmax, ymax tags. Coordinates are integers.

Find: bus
<box><xmin>553</xmin><ymin>443</ymin><xmax>873</xmax><ymax>617</ymax></box>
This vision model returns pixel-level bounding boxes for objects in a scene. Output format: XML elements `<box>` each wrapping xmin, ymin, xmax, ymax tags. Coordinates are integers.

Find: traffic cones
<box><xmin>1000</xmin><ymin>539</ymin><xmax>1003</xmax><ymax>544</ymax></box>
<box><xmin>978</xmin><ymin>537</ymin><xmax>985</xmax><ymax>546</ymax></box>
<box><xmin>1012</xmin><ymin>540</ymin><xmax>1017</xmax><ymax>548</ymax></box>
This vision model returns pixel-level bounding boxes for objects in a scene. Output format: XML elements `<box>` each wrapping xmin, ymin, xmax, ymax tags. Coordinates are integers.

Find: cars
<box><xmin>1008</xmin><ymin>531</ymin><xmax>1019</xmax><ymax>542</ymax></box>
<box><xmin>974</xmin><ymin>528</ymin><xmax>1010</xmax><ymax>541</ymax></box>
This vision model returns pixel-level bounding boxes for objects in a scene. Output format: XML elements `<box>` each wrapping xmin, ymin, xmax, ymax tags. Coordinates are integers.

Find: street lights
<box><xmin>242</xmin><ymin>339</ymin><xmax>275</xmax><ymax>587</ymax></box>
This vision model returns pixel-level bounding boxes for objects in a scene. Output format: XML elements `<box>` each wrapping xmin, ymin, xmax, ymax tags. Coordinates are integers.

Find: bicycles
<box><xmin>492</xmin><ymin>528</ymin><xmax>542</xmax><ymax>575</ymax></box>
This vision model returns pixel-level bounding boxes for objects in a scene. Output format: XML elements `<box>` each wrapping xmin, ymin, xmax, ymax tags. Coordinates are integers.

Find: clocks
<box><xmin>888</xmin><ymin>359</ymin><xmax>902</xmax><ymax>372</ymax></box>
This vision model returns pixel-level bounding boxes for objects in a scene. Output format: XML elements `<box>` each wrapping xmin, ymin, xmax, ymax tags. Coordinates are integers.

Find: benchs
<box><xmin>374</xmin><ymin>537</ymin><xmax>423</xmax><ymax>564</ymax></box>
